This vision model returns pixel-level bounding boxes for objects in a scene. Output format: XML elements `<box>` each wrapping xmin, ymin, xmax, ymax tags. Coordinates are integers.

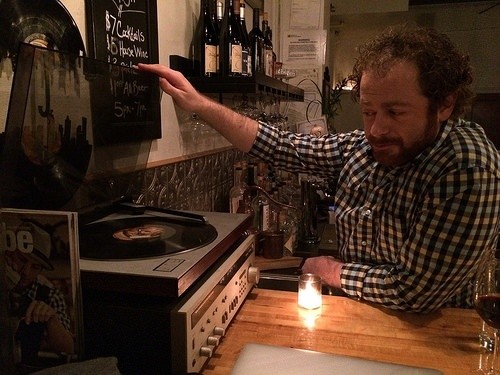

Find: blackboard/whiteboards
<box><xmin>86</xmin><ymin>0</ymin><xmax>163</xmax><ymax>143</ymax></box>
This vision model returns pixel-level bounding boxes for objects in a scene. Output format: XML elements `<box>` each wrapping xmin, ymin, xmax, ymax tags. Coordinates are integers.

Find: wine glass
<box><xmin>472</xmin><ymin>246</ymin><xmax>500</xmax><ymax>375</ymax></box>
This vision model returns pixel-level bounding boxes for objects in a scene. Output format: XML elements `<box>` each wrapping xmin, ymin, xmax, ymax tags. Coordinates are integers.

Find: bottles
<box><xmin>188</xmin><ymin>0</ymin><xmax>276</xmax><ymax>79</ymax></box>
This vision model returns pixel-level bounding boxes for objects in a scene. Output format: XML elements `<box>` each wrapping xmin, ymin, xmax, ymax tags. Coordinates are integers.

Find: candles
<box><xmin>298</xmin><ymin>277</ymin><xmax>321</xmax><ymax>310</ymax></box>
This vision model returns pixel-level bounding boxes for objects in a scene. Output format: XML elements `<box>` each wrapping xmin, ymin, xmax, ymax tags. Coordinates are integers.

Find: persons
<box><xmin>6</xmin><ymin>222</ymin><xmax>75</xmax><ymax>362</ymax></box>
<box><xmin>138</xmin><ymin>25</ymin><xmax>500</xmax><ymax>314</ymax></box>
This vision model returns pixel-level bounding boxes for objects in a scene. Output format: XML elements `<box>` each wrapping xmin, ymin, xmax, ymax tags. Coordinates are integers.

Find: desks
<box><xmin>196</xmin><ymin>288</ymin><xmax>500</xmax><ymax>375</ymax></box>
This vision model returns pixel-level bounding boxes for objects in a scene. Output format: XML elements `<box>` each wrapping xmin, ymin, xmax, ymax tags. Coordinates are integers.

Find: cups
<box><xmin>297</xmin><ymin>272</ymin><xmax>323</xmax><ymax>311</ymax></box>
